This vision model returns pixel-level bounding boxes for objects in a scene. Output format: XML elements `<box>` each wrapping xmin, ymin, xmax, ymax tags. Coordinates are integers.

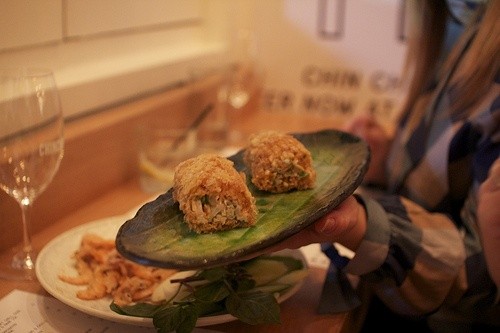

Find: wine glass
<box><xmin>0</xmin><ymin>66</ymin><xmax>64</xmax><ymax>281</ymax></box>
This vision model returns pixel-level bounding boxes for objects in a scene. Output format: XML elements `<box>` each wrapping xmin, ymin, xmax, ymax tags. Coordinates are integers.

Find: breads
<box><xmin>244</xmin><ymin>130</ymin><xmax>316</xmax><ymax>193</ymax></box>
<box><xmin>172</xmin><ymin>154</ymin><xmax>257</xmax><ymax>233</ymax></box>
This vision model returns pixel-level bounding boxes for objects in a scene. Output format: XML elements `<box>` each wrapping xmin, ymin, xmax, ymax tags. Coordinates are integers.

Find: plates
<box><xmin>116</xmin><ymin>128</ymin><xmax>373</xmax><ymax>271</ymax></box>
<box><xmin>35</xmin><ymin>214</ymin><xmax>310</xmax><ymax>326</ymax></box>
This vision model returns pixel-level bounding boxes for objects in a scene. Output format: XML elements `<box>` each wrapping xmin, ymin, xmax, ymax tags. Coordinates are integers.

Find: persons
<box><xmin>204</xmin><ymin>0</ymin><xmax>500</xmax><ymax>333</ymax></box>
